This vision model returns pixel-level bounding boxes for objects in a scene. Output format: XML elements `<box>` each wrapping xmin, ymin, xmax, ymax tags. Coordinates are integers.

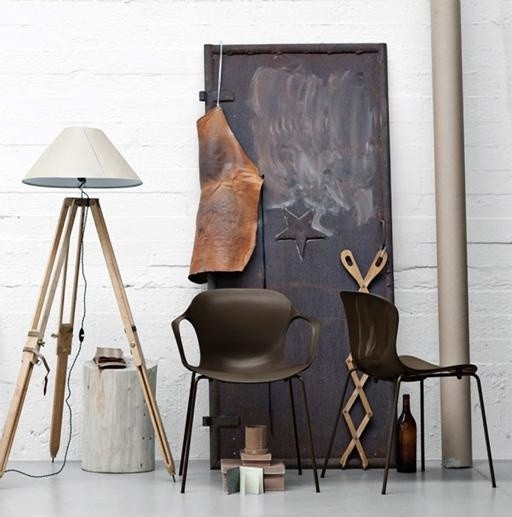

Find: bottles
<box><xmin>395</xmin><ymin>393</ymin><xmax>418</xmax><ymax>473</ymax></box>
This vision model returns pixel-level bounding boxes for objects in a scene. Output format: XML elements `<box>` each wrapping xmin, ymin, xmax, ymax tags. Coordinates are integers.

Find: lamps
<box><xmin>0</xmin><ymin>124</ymin><xmax>177</xmax><ymax>489</ymax></box>
